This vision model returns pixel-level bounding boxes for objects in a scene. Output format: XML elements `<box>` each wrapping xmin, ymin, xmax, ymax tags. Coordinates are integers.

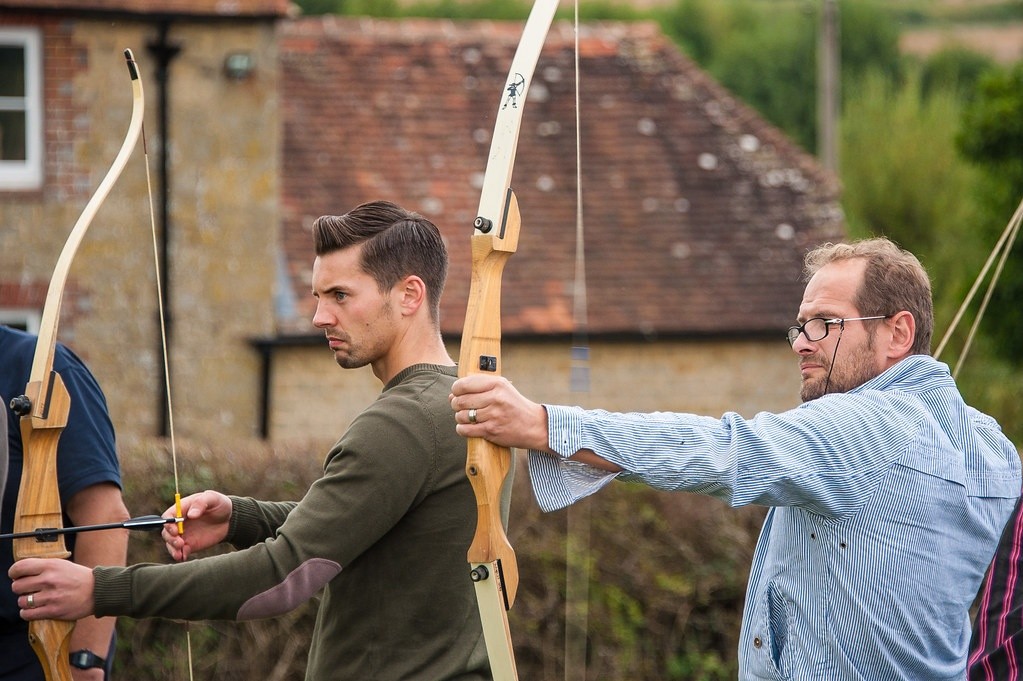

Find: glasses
<box><xmin>785</xmin><ymin>313</ymin><xmax>896</xmax><ymax>349</ymax></box>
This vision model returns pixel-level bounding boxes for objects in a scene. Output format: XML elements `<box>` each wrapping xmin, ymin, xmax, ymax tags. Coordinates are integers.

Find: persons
<box><xmin>0</xmin><ymin>325</ymin><xmax>131</xmax><ymax>681</ymax></box>
<box><xmin>965</xmin><ymin>496</ymin><xmax>1023</xmax><ymax>681</ymax></box>
<box><xmin>447</xmin><ymin>238</ymin><xmax>1022</xmax><ymax>681</ymax></box>
<box><xmin>9</xmin><ymin>199</ymin><xmax>515</xmax><ymax>681</ymax></box>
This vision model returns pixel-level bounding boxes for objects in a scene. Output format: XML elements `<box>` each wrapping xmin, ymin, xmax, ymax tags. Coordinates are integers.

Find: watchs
<box><xmin>69</xmin><ymin>648</ymin><xmax>107</xmax><ymax>672</ymax></box>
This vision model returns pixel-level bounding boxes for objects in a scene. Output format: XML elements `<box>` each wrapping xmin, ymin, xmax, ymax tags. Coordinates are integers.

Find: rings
<box><xmin>28</xmin><ymin>595</ymin><xmax>35</xmax><ymax>609</ymax></box>
<box><xmin>469</xmin><ymin>409</ymin><xmax>477</xmax><ymax>424</ymax></box>
